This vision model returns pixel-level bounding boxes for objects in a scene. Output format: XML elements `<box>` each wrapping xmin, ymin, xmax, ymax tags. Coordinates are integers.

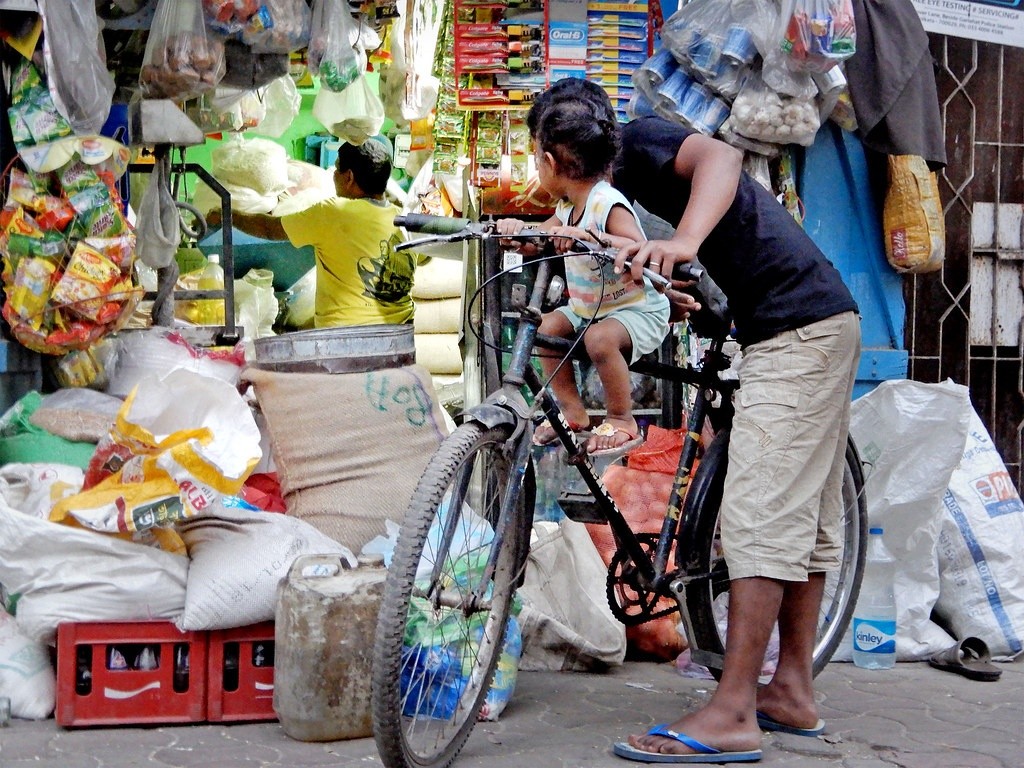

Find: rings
<box><xmin>649</xmin><ymin>261</ymin><xmax>661</xmax><ymax>267</ymax></box>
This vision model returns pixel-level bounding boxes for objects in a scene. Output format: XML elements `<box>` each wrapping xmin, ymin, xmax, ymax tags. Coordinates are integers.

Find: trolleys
<box><xmin>124</xmin><ymin>162</ymin><xmax>244</xmax><ymax>347</ymax></box>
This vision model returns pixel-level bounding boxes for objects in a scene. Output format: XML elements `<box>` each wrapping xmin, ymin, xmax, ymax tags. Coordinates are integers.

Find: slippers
<box><xmin>756</xmin><ymin>711</ymin><xmax>825</xmax><ymax>736</ymax></box>
<box><xmin>587</xmin><ymin>423</ymin><xmax>643</xmax><ymax>457</ymax></box>
<box><xmin>929</xmin><ymin>636</ymin><xmax>1002</xmax><ymax>682</ymax></box>
<box><xmin>533</xmin><ymin>414</ymin><xmax>592</xmax><ymax>446</ymax></box>
<box><xmin>614</xmin><ymin>724</ymin><xmax>763</xmax><ymax>762</ymax></box>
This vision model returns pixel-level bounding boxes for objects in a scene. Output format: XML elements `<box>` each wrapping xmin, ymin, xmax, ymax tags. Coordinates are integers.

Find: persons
<box><xmin>494</xmin><ymin>96</ymin><xmax>672</xmax><ymax>456</ymax></box>
<box><xmin>525</xmin><ymin>76</ymin><xmax>862</xmax><ymax>763</ymax></box>
<box><xmin>205</xmin><ymin>136</ymin><xmax>433</xmax><ymax>336</ymax></box>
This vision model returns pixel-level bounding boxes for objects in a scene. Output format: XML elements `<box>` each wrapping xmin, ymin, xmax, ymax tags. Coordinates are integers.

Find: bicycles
<box><xmin>363</xmin><ymin>213</ymin><xmax>873</xmax><ymax>768</ymax></box>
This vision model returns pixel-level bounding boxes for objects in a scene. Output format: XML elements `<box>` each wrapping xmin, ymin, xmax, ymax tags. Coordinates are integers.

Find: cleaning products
<box><xmin>1</xmin><ymin>36</ymin><xmax>142</xmax><ymax>387</ymax></box>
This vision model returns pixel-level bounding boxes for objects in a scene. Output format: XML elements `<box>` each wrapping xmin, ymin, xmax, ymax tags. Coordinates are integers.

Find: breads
<box><xmin>141</xmin><ymin>29</ymin><xmax>225</xmax><ymax>98</ymax></box>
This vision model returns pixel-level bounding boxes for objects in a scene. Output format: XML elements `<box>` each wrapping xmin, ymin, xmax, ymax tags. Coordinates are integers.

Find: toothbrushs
<box><xmin>458</xmin><ymin>17</ymin><xmax>547</xmax><ymax>106</ymax></box>
<box><xmin>587</xmin><ymin>13</ymin><xmax>649</xmax><ymax>124</ymax></box>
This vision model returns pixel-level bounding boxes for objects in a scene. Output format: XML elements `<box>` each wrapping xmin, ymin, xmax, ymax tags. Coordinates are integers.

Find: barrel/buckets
<box><xmin>271</xmin><ymin>552</ymin><xmax>393</xmax><ymax>742</ymax></box>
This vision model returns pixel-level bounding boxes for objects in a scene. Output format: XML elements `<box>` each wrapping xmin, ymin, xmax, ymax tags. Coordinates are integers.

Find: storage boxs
<box><xmin>53</xmin><ymin>621</ymin><xmax>208</xmax><ymax>730</ymax></box>
<box><xmin>209</xmin><ymin>622</ymin><xmax>277</xmax><ymax>722</ymax></box>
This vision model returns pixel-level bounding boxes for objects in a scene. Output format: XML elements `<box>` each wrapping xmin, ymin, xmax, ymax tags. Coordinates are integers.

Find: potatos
<box><xmin>583</xmin><ymin>466</ymin><xmax>691</xmax><ymax>657</ymax></box>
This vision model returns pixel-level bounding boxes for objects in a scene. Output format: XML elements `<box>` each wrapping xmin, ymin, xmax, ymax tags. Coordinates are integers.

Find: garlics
<box><xmin>730</xmin><ymin>92</ymin><xmax>819</xmax><ymax>149</ymax></box>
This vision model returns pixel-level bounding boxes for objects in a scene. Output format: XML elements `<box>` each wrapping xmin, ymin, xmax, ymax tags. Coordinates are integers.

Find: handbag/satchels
<box><xmin>628</xmin><ymin>0</ymin><xmax>857</xmax><ymax>156</ymax></box>
<box><xmin>138</xmin><ymin>1</ymin><xmax>385</xmax><ymax>145</ymax></box>
<box><xmin>515</xmin><ymin>517</ymin><xmax>627</xmax><ymax>671</ymax></box>
<box><xmin>883</xmin><ymin>150</ymin><xmax>944</xmax><ymax>277</ymax></box>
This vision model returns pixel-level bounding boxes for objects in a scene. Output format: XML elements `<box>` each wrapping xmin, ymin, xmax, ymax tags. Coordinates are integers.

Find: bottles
<box><xmin>851</xmin><ymin>528</ymin><xmax>898</xmax><ymax>669</ymax></box>
<box><xmin>529</xmin><ymin>447</ymin><xmax>580</xmax><ymax>524</ymax></box>
<box><xmin>502</xmin><ymin>325</ymin><xmax>546</xmax><ymax>410</ymax></box>
<box><xmin>70</xmin><ymin>636</ymin><xmax>275</xmax><ymax>703</ymax></box>
<box><xmin>196</xmin><ymin>254</ymin><xmax>225</xmax><ymax>325</ymax></box>
